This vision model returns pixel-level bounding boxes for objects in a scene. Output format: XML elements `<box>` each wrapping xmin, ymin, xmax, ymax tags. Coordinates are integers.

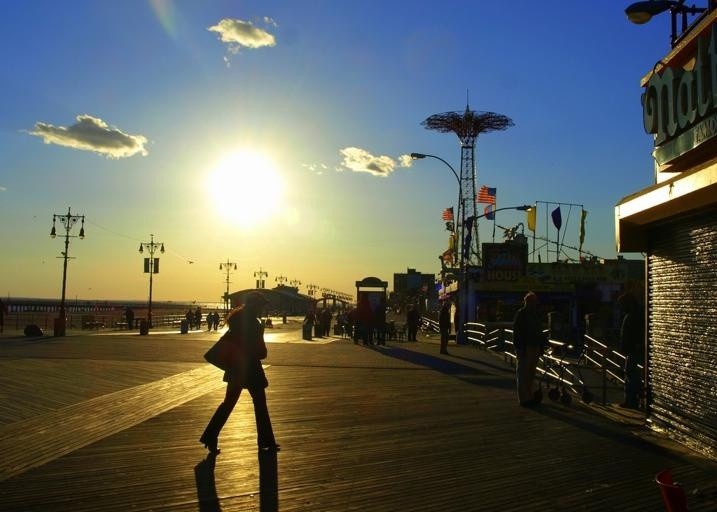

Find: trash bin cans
<box><xmin>303</xmin><ymin>325</ymin><xmax>312</xmax><ymax>340</ymax></box>
<box><xmin>334</xmin><ymin>325</ymin><xmax>341</xmax><ymax>335</ymax></box>
<box><xmin>314</xmin><ymin>325</ymin><xmax>323</xmax><ymax>337</ymax></box>
<box><xmin>54</xmin><ymin>318</ymin><xmax>66</xmax><ymax>337</ymax></box>
<box><xmin>140</xmin><ymin>321</ymin><xmax>150</xmax><ymax>335</ymax></box>
<box><xmin>181</xmin><ymin>319</ymin><xmax>189</xmax><ymax>334</ymax></box>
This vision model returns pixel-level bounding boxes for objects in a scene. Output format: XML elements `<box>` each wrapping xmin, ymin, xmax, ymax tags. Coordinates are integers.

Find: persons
<box><xmin>194</xmin><ymin>307</ymin><xmax>202</xmax><ymax>329</ymax></box>
<box><xmin>185</xmin><ymin>308</ymin><xmax>194</xmax><ymax>330</ymax></box>
<box><xmin>510</xmin><ymin>290</ymin><xmax>550</xmax><ymax>409</ymax></box>
<box><xmin>302</xmin><ymin>304</ymin><xmax>422</xmax><ymax>346</ymax></box>
<box><xmin>206</xmin><ymin>311</ymin><xmax>213</xmax><ymax>330</ymax></box>
<box><xmin>438</xmin><ymin>301</ymin><xmax>452</xmax><ymax>355</ymax></box>
<box><xmin>615</xmin><ymin>292</ymin><xmax>645</xmax><ymax>410</ymax></box>
<box><xmin>125</xmin><ymin>308</ymin><xmax>134</xmax><ymax>330</ymax></box>
<box><xmin>213</xmin><ymin>311</ymin><xmax>219</xmax><ymax>330</ymax></box>
<box><xmin>0</xmin><ymin>298</ymin><xmax>8</xmax><ymax>332</ymax></box>
<box><xmin>197</xmin><ymin>290</ymin><xmax>281</xmax><ymax>454</ymax></box>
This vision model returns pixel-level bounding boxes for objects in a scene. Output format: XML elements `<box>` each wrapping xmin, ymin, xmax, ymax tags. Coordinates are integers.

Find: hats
<box><xmin>247</xmin><ymin>292</ymin><xmax>272</xmax><ymax>305</ymax></box>
<box><xmin>525</xmin><ymin>293</ymin><xmax>540</xmax><ymax>304</ymax></box>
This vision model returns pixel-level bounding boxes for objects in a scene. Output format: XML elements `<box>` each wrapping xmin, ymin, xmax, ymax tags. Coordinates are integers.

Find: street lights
<box><xmin>218</xmin><ymin>255</ymin><xmax>239</xmax><ymax>329</ymax></box>
<box><xmin>138</xmin><ymin>232</ymin><xmax>167</xmax><ymax>329</ymax></box>
<box><xmin>254</xmin><ymin>268</ymin><xmax>353</xmax><ymax>325</ymax></box>
<box><xmin>408</xmin><ymin>150</ymin><xmax>535</xmax><ymax>347</ymax></box>
<box><xmin>50</xmin><ymin>206</ymin><xmax>86</xmax><ymax>336</ymax></box>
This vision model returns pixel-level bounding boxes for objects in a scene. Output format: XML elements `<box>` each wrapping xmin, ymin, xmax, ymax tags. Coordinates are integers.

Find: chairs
<box><xmin>386</xmin><ymin>320</ymin><xmax>408</xmax><ymax>342</ymax></box>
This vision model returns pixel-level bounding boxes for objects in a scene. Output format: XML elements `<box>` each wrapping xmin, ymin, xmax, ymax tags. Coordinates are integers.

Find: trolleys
<box><xmin>532</xmin><ymin>328</ymin><xmax>599</xmax><ymax>408</ymax></box>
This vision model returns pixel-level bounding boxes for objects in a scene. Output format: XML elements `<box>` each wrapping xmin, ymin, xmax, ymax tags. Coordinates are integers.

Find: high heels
<box><xmin>258</xmin><ymin>444</ymin><xmax>280</xmax><ymax>451</ymax></box>
<box><xmin>205</xmin><ymin>444</ymin><xmax>220</xmax><ymax>453</ymax></box>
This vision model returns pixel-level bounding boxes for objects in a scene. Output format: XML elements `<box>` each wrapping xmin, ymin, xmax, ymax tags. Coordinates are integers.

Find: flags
<box><xmin>442</xmin><ymin>207</ymin><xmax>452</xmax><ymax>221</ymax></box>
<box><xmin>475</xmin><ymin>186</ymin><xmax>495</xmax><ymax>205</ymax></box>
<box><xmin>483</xmin><ymin>204</ymin><xmax>495</xmax><ymax>221</ymax></box>
<box><xmin>578</xmin><ymin>209</ymin><xmax>588</xmax><ymax>249</ymax></box>
<box><xmin>550</xmin><ymin>207</ymin><xmax>561</xmax><ymax>230</ymax></box>
<box><xmin>527</xmin><ymin>207</ymin><xmax>535</xmax><ymax>231</ymax></box>
<box><xmin>445</xmin><ymin>222</ymin><xmax>453</xmax><ymax>232</ymax></box>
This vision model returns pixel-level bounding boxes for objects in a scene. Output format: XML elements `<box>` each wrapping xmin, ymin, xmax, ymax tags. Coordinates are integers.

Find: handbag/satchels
<box><xmin>205</xmin><ymin>330</ymin><xmax>234</xmax><ymax>370</ymax></box>
